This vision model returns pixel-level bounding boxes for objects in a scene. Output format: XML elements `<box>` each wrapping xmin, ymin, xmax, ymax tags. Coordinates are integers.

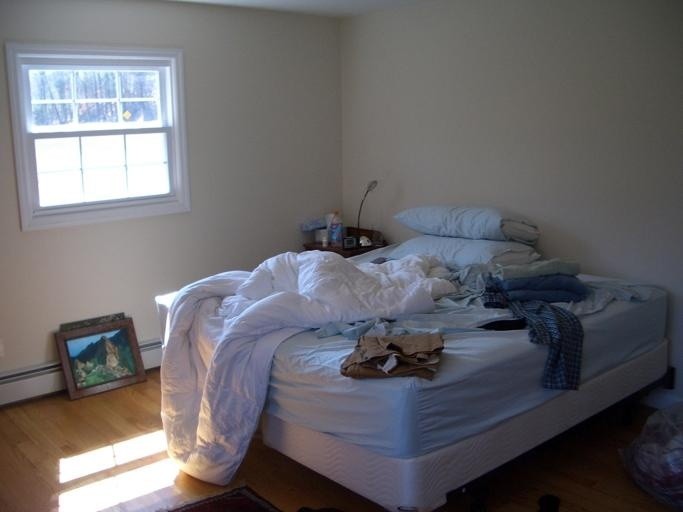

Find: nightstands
<box><xmin>304</xmin><ymin>243</ymin><xmax>386</xmax><ymax>258</ymax></box>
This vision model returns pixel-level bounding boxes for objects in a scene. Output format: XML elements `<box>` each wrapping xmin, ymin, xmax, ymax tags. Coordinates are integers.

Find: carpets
<box><xmin>166</xmin><ymin>485</ymin><xmax>283</xmax><ymax>512</ymax></box>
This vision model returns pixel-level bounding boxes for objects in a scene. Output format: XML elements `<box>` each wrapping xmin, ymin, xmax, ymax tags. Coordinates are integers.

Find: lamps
<box><xmin>356</xmin><ymin>180</ymin><xmax>378</xmax><ymax>247</ymax></box>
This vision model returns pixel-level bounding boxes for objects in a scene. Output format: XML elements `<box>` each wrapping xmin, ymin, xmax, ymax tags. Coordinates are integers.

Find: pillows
<box><xmin>389</xmin><ymin>205</ymin><xmax>543</xmax><ymax>272</ymax></box>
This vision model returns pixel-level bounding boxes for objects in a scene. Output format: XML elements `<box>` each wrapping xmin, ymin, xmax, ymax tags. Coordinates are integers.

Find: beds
<box><xmin>159</xmin><ymin>257</ymin><xmax>669</xmax><ymax>512</ymax></box>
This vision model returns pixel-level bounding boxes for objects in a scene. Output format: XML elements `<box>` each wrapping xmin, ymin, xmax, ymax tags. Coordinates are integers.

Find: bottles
<box><xmin>322</xmin><ymin>211</ymin><xmax>343</xmax><ymax>248</ymax></box>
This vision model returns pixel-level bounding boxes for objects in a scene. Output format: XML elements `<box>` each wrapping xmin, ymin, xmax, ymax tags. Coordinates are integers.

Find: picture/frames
<box><xmin>55</xmin><ymin>317</ymin><xmax>147</xmax><ymax>401</ymax></box>
